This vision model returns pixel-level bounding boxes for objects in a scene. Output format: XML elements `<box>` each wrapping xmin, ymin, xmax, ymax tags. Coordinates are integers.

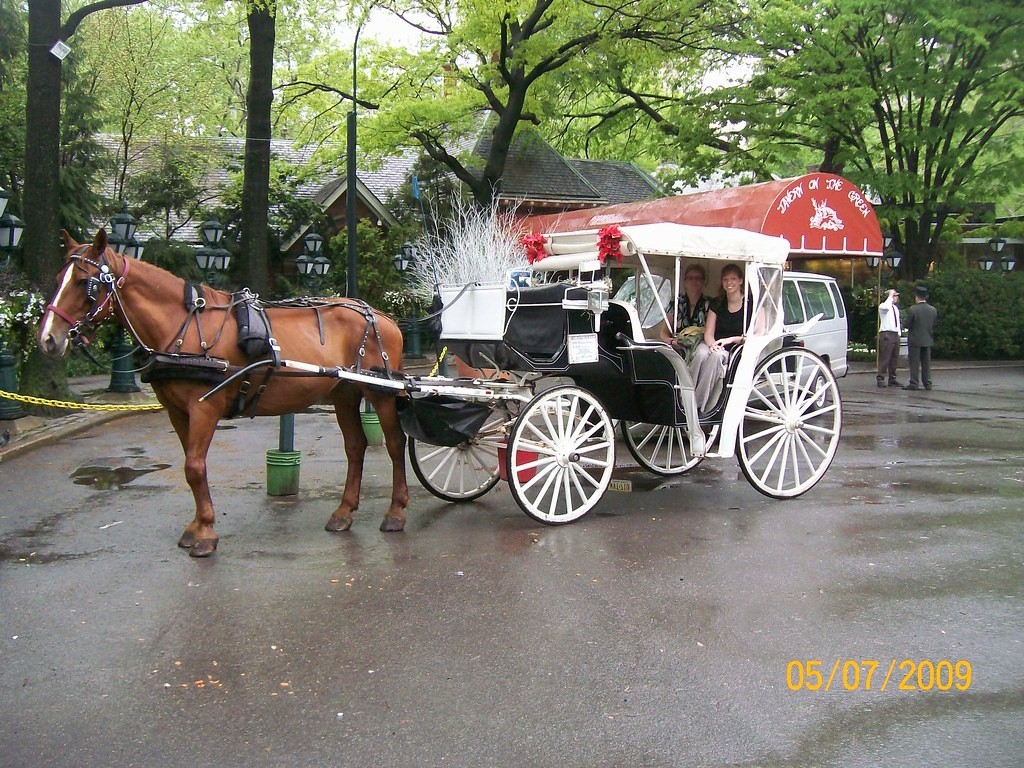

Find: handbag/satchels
<box><xmin>678</xmin><ymin>325</ymin><xmax>706</xmax><ymax>362</ymax></box>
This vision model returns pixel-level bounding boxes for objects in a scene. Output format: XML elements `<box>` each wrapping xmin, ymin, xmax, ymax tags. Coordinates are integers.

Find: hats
<box><xmin>915</xmin><ymin>286</ymin><xmax>929</xmax><ymax>297</ymax></box>
<box><xmin>887</xmin><ymin>289</ymin><xmax>900</xmax><ymax>296</ymax></box>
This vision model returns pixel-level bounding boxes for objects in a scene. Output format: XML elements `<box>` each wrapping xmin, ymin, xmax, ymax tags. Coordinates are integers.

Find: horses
<box><xmin>38</xmin><ymin>228</ymin><xmax>408</xmax><ymax>556</ymax></box>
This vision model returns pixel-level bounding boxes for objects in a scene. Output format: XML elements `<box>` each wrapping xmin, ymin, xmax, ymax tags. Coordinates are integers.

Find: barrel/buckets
<box><xmin>265</xmin><ymin>448</ymin><xmax>302</xmax><ymax>496</ymax></box>
<box><xmin>360</xmin><ymin>412</ymin><xmax>383</xmax><ymax>446</ymax></box>
<box><xmin>496</xmin><ymin>440</ymin><xmax>539</xmax><ymax>483</ymax></box>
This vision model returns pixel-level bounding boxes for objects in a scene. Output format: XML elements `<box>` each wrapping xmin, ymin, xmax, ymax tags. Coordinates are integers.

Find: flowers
<box><xmin>521</xmin><ymin>234</ymin><xmax>547</xmax><ymax>267</ymax></box>
<box><xmin>596</xmin><ymin>223</ymin><xmax>623</xmax><ymax>268</ymax></box>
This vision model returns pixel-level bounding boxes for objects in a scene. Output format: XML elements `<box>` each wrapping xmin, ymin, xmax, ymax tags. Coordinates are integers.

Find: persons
<box><xmin>876</xmin><ymin>288</ymin><xmax>904</xmax><ymax>388</ymax></box>
<box><xmin>901</xmin><ymin>286</ymin><xmax>937</xmax><ymax>390</ymax></box>
<box><xmin>661</xmin><ymin>265</ymin><xmax>714</xmax><ymax>360</ymax></box>
<box><xmin>686</xmin><ymin>263</ymin><xmax>765</xmax><ymax>416</ymax></box>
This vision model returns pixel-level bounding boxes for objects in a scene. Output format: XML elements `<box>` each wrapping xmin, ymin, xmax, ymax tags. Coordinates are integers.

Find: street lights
<box><xmin>864</xmin><ymin>246</ymin><xmax>904</xmax><ymax>353</ymax></box>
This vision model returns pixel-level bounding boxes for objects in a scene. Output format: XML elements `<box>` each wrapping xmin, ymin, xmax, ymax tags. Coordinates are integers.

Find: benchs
<box><xmin>426</xmin><ymin>282</ymin><xmax>592</xmax><ymax>360</ymax></box>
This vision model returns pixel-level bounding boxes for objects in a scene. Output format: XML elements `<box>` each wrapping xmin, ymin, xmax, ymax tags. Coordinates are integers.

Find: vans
<box><xmin>744</xmin><ymin>268</ymin><xmax>850</xmax><ymax>417</ymax></box>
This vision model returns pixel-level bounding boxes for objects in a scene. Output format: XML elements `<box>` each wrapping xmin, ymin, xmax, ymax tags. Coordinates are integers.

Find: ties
<box><xmin>892</xmin><ymin>305</ymin><xmax>897</xmax><ymax>327</ymax></box>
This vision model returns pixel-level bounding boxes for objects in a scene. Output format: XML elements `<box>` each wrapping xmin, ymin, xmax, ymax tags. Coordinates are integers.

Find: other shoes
<box><xmin>901</xmin><ymin>384</ymin><xmax>918</xmax><ymax>390</ymax></box>
<box><xmin>877</xmin><ymin>381</ymin><xmax>887</xmax><ymax>387</ymax></box>
<box><xmin>924</xmin><ymin>385</ymin><xmax>932</xmax><ymax>390</ymax></box>
<box><xmin>888</xmin><ymin>381</ymin><xmax>904</xmax><ymax>387</ymax></box>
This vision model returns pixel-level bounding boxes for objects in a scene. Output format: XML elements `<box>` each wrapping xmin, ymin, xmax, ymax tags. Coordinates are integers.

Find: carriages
<box><xmin>36</xmin><ymin>219</ymin><xmax>845</xmax><ymax>559</ymax></box>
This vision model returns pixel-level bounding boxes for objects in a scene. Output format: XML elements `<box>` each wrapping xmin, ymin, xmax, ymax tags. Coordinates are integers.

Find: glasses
<box><xmin>685</xmin><ymin>276</ymin><xmax>704</xmax><ymax>282</ymax></box>
